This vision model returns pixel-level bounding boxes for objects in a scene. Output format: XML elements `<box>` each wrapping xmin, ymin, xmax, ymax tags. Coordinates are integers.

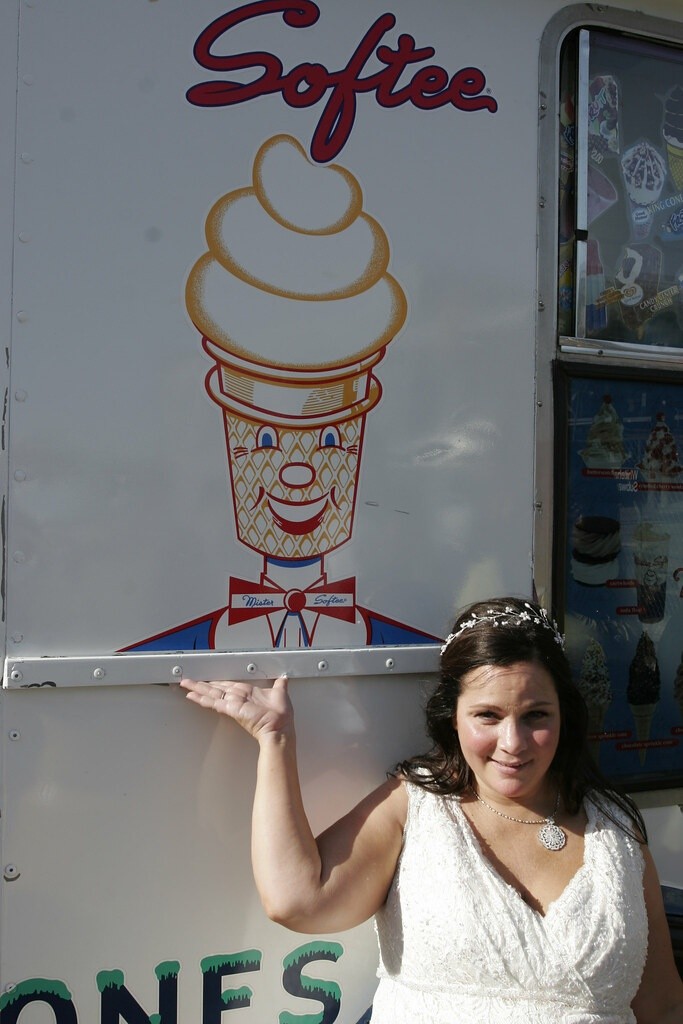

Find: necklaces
<box><xmin>470</xmin><ymin>785</ymin><xmax>565</xmax><ymax>850</ymax></box>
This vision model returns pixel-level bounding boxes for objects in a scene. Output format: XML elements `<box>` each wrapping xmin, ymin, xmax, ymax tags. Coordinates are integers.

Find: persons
<box><xmin>181</xmin><ymin>596</ymin><xmax>683</xmax><ymax>1024</ymax></box>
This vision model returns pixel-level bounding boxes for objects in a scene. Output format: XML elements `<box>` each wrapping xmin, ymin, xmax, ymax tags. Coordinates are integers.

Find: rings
<box><xmin>221</xmin><ymin>692</ymin><xmax>226</xmax><ymax>699</ymax></box>
